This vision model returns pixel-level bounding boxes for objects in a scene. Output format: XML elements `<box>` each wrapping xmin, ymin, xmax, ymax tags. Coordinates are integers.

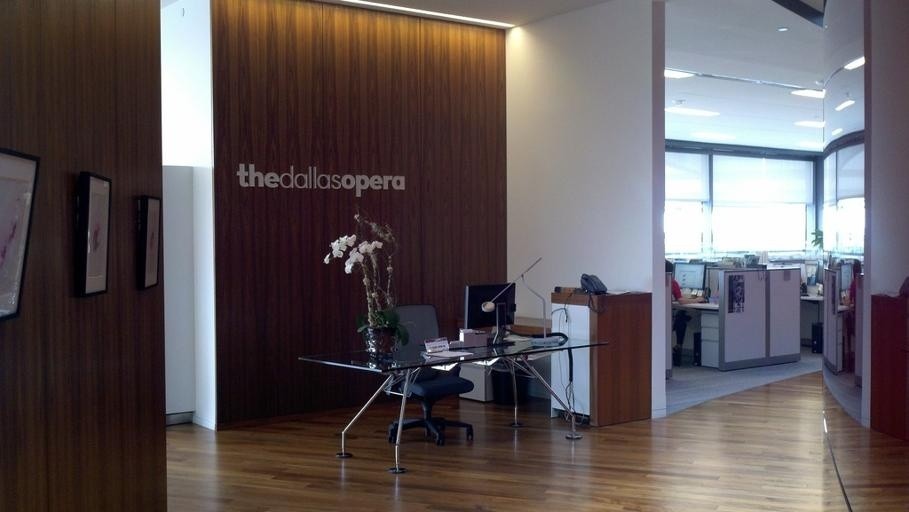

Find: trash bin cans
<box><xmin>491</xmin><ymin>362</ymin><xmax>532</xmax><ymax>406</ymax></box>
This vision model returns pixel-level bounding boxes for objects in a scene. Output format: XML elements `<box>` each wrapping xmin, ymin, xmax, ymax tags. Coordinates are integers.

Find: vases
<box><xmin>365</xmin><ymin>329</ymin><xmax>399</xmax><ymax>354</ymax></box>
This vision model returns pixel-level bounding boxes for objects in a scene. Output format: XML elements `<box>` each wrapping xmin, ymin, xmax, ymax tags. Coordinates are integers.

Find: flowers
<box><xmin>323</xmin><ymin>214</ymin><xmax>398</xmax><ymax>328</ymax></box>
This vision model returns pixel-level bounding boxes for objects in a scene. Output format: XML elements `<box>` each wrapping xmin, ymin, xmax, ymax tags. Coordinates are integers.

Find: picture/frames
<box><xmin>134</xmin><ymin>195</ymin><xmax>162</xmax><ymax>291</ymax></box>
<box><xmin>73</xmin><ymin>171</ymin><xmax>112</xmax><ymax>298</ymax></box>
<box><xmin>0</xmin><ymin>147</ymin><xmax>40</xmax><ymax>320</ymax></box>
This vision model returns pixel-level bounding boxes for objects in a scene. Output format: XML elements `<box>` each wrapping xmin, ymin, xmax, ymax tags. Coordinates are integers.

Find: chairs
<box><xmin>386</xmin><ymin>303</ymin><xmax>474</xmax><ymax>446</ymax></box>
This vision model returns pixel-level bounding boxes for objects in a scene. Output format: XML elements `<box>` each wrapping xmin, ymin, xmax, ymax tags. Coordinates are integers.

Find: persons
<box><xmin>665</xmin><ymin>259</ymin><xmax>706</xmax><ymax>367</ymax></box>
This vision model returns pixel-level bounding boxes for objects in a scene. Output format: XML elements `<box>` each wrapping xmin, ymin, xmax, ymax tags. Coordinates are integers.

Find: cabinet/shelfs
<box><xmin>550</xmin><ymin>286</ymin><xmax>652</xmax><ymax>429</ymax></box>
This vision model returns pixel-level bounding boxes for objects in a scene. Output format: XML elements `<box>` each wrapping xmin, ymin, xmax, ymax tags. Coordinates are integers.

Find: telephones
<box><xmin>581</xmin><ymin>273</ymin><xmax>607</xmax><ymax>294</ymax></box>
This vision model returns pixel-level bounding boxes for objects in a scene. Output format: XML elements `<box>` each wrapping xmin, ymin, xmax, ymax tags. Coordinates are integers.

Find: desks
<box><xmin>801</xmin><ymin>295</ymin><xmax>824</xmax><ymax>354</ymax></box>
<box><xmin>298</xmin><ymin>335</ymin><xmax>609</xmax><ymax>476</ymax></box>
<box><xmin>672</xmin><ymin>301</ymin><xmax>719</xmax><ymax>368</ymax></box>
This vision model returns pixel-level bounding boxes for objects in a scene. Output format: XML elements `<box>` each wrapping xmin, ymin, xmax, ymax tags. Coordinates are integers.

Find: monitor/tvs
<box><xmin>840</xmin><ymin>264</ymin><xmax>853</xmax><ymax>291</ymax></box>
<box><xmin>673</xmin><ymin>262</ymin><xmax>706</xmax><ymax>290</ymax></box>
<box><xmin>464</xmin><ymin>282</ymin><xmax>517</xmax><ymax>347</ymax></box>
